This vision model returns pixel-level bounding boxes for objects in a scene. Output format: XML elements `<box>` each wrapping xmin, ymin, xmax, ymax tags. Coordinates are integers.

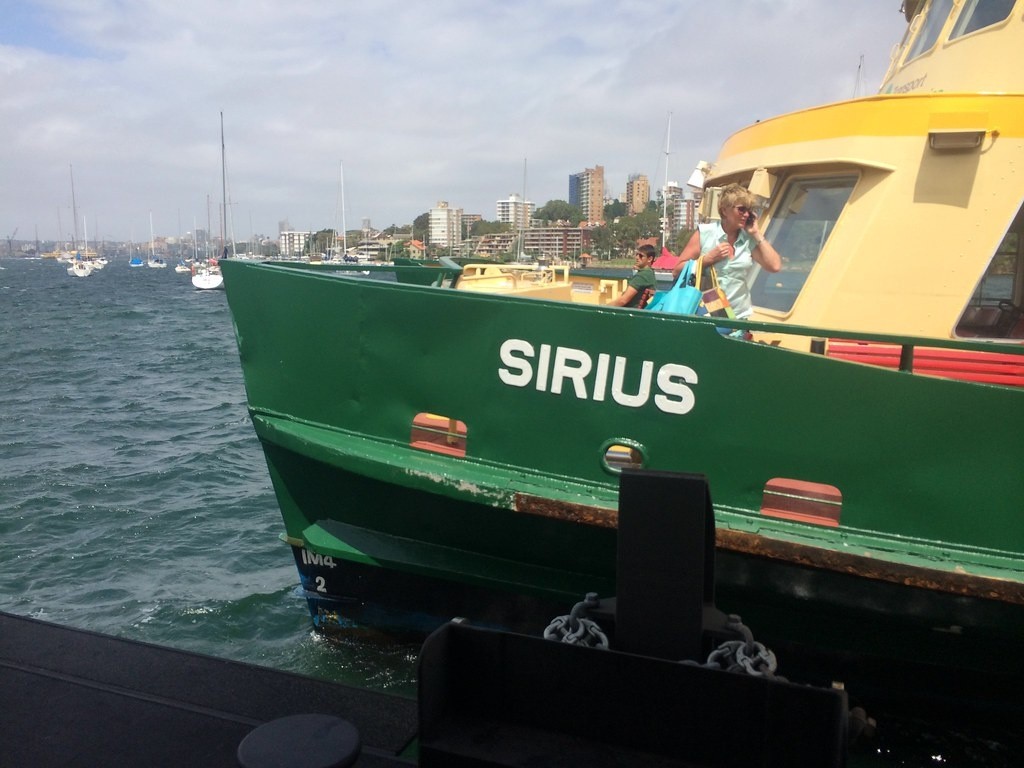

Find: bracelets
<box><xmin>757</xmin><ymin>237</ymin><xmax>764</xmax><ymax>245</ymax></box>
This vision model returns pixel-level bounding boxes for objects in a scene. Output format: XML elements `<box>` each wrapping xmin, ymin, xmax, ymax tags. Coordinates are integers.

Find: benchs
<box><xmin>825</xmin><ymin>332</ymin><xmax>1024</xmax><ymax>393</ymax></box>
<box><xmin>636</xmin><ymin>288</ymin><xmax>655</xmax><ymax>308</ymax></box>
<box><xmin>418</xmin><ymin>617</ymin><xmax>850</xmax><ymax>768</ymax></box>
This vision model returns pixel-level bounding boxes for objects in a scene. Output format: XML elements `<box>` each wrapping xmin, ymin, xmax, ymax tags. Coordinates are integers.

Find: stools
<box><xmin>234</xmin><ymin>714</ymin><xmax>362</xmax><ymax>768</ymax></box>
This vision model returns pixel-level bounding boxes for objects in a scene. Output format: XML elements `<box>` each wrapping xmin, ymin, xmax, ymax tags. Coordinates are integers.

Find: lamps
<box><xmin>687</xmin><ymin>160</ymin><xmax>709</xmax><ymax>190</ymax></box>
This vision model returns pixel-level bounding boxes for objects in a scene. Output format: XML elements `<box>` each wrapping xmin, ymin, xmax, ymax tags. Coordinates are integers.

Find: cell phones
<box><xmin>746</xmin><ymin>215</ymin><xmax>754</xmax><ymax>225</ymax></box>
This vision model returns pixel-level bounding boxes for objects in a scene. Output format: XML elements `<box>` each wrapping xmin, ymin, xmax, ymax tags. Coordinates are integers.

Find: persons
<box><xmin>607</xmin><ymin>245</ymin><xmax>657</xmax><ymax>309</ymax></box>
<box><xmin>672</xmin><ymin>183</ymin><xmax>782</xmax><ymax>341</ymax></box>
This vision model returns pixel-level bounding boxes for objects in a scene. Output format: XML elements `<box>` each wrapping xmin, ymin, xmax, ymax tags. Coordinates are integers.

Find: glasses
<box><xmin>734</xmin><ymin>206</ymin><xmax>752</xmax><ymax>215</ymax></box>
<box><xmin>635</xmin><ymin>253</ymin><xmax>646</xmax><ymax>259</ymax></box>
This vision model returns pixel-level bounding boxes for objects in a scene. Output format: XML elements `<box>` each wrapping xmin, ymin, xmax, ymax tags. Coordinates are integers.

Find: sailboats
<box><xmin>126</xmin><ymin>109</ymin><xmax>394</xmax><ymax>289</ymax></box>
<box><xmin>54</xmin><ymin>111</ymin><xmax>108</xmax><ymax>278</ymax></box>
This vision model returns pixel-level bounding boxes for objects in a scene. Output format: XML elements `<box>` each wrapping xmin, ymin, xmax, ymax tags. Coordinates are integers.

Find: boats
<box><xmin>220</xmin><ymin>1</ymin><xmax>1022</xmax><ymax>759</ymax></box>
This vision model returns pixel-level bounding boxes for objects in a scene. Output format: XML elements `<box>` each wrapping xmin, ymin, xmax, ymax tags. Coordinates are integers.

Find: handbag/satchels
<box><xmin>643</xmin><ymin>255</ymin><xmax>741</xmax><ymax>334</ymax></box>
